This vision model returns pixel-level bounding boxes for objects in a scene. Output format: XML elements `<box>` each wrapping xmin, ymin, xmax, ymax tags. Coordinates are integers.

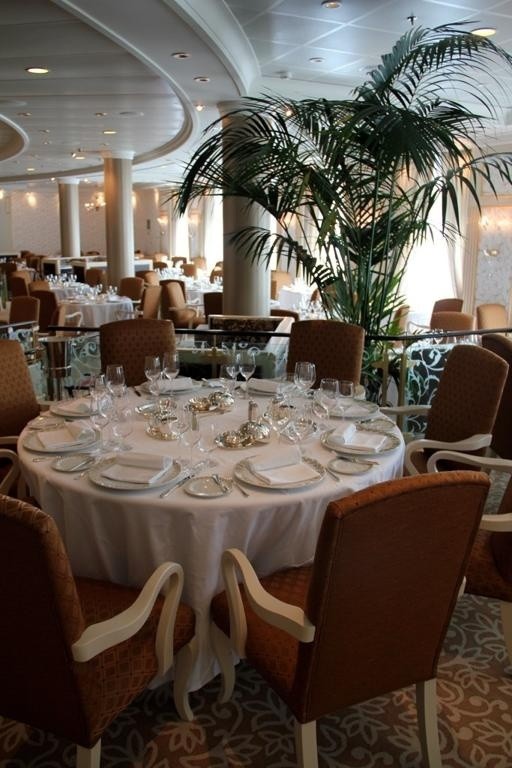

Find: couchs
<box><xmin>175</xmin><ymin>314</ymin><xmax>295</xmax><ymax>382</ymax></box>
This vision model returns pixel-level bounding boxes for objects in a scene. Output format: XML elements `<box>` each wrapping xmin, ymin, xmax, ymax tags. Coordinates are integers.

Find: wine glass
<box><xmin>224</xmin><ymin>350</ymin><xmax>257</xmax><ymax>401</ymax></box>
<box><xmin>434</xmin><ymin>328</ymin><xmax>444</xmax><ymax>345</ymax></box>
<box><xmin>88</xmin><ymin>363</ymin><xmax>134</xmax><ymax>451</ymax></box>
<box><xmin>163</xmin><ymin>399</ymin><xmax>222</xmax><ymax>476</ymax></box>
<box><xmin>143</xmin><ymin>350</ymin><xmax>181</xmax><ymax>402</ymax></box>
<box><xmin>268</xmin><ymin>360</ymin><xmax>355</xmax><ymax>451</ymax></box>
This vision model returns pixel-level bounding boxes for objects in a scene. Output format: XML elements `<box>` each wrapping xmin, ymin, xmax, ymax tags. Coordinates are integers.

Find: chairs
<box><xmin>2</xmin><ymin>337</ymin><xmax>51</xmax><ymax>455</ymax></box>
<box><xmin>481</xmin><ymin>333</ymin><xmax>509</xmax><ymax>362</ymax></box>
<box><xmin>428</xmin><ymin>447</ymin><xmax>511</xmax><ymax>666</ymax></box>
<box><xmin>285</xmin><ymin>320</ymin><xmax>366</xmax><ymax>402</ymax></box>
<box><xmin>207</xmin><ymin>470</ymin><xmax>492</xmax><ymax>766</ymax></box>
<box><xmin>389</xmin><ymin>297</ymin><xmax>511</xmax><ymax>345</ymax></box>
<box><xmin>380</xmin><ymin>341</ymin><xmax>509</xmax><ymax>474</ymax></box>
<box><xmin>97</xmin><ymin>319</ymin><xmax>177</xmax><ymax>388</ymax></box>
<box><xmin>1</xmin><ymin>250</ymin><xmax>332</xmax><ymax>336</ymax></box>
<box><xmin>1</xmin><ymin>447</ymin><xmax>198</xmax><ymax>766</ymax></box>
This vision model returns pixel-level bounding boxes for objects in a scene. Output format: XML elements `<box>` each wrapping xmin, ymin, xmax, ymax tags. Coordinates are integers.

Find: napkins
<box><xmin>327</xmin><ymin>421</ymin><xmax>387</xmax><ymax>453</ymax></box>
<box><xmin>59</xmin><ymin>394</ymin><xmax>109</xmax><ymax>414</ymax></box>
<box><xmin>246</xmin><ymin>378</ymin><xmax>283</xmax><ymax>393</ymax></box>
<box><xmin>36</xmin><ymin>418</ymin><xmax>96</xmax><ymax>450</ymax></box>
<box><xmin>244</xmin><ymin>444</ymin><xmax>322</xmax><ymax>486</ymax></box>
<box><xmin>326</xmin><ymin>398</ymin><xmax>370</xmax><ymax>416</ymax></box>
<box><xmin>100</xmin><ymin>453</ymin><xmax>175</xmax><ymax>484</ymax></box>
<box><xmin>156</xmin><ymin>376</ymin><xmax>195</xmax><ymax>391</ymax></box>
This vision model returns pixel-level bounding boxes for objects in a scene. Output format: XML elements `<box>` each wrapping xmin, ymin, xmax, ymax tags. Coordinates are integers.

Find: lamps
<box><xmin>478</xmin><ymin>213</ymin><xmax>511</xmax><ymax>256</ymax></box>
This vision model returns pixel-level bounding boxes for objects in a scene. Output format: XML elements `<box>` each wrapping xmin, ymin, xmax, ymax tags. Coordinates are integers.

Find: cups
<box><xmin>75</xmin><ymin>283</ymin><xmax>118</xmax><ymax>298</ymax></box>
<box><xmin>46</xmin><ymin>273</ymin><xmax>78</xmax><ymax>287</ymax></box>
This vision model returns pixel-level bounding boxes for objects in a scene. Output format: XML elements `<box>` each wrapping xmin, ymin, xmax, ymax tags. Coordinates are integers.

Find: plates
<box><xmin>50</xmin><ymin>451</ymin><xmax>182</xmax><ymax>492</ymax></box>
<box><xmin>359</xmin><ymin>418</ymin><xmax>396</xmax><ymax>432</ymax></box>
<box><xmin>330</xmin><ymin>396</ymin><xmax>380</xmax><ymax>419</ymax></box>
<box><xmin>49</xmin><ymin>398</ymin><xmax>91</xmax><ymax>417</ymax></box>
<box><xmin>319</xmin><ymin>424</ymin><xmax>402</xmax><ymax>475</ymax></box>
<box><xmin>183</xmin><ymin>475</ymin><xmax>234</xmax><ymax>498</ymax></box>
<box><xmin>21</xmin><ymin>415</ymin><xmax>104</xmax><ymax>452</ymax></box>
<box><xmin>233</xmin><ymin>451</ymin><xmax>328</xmax><ymax>490</ymax></box>
